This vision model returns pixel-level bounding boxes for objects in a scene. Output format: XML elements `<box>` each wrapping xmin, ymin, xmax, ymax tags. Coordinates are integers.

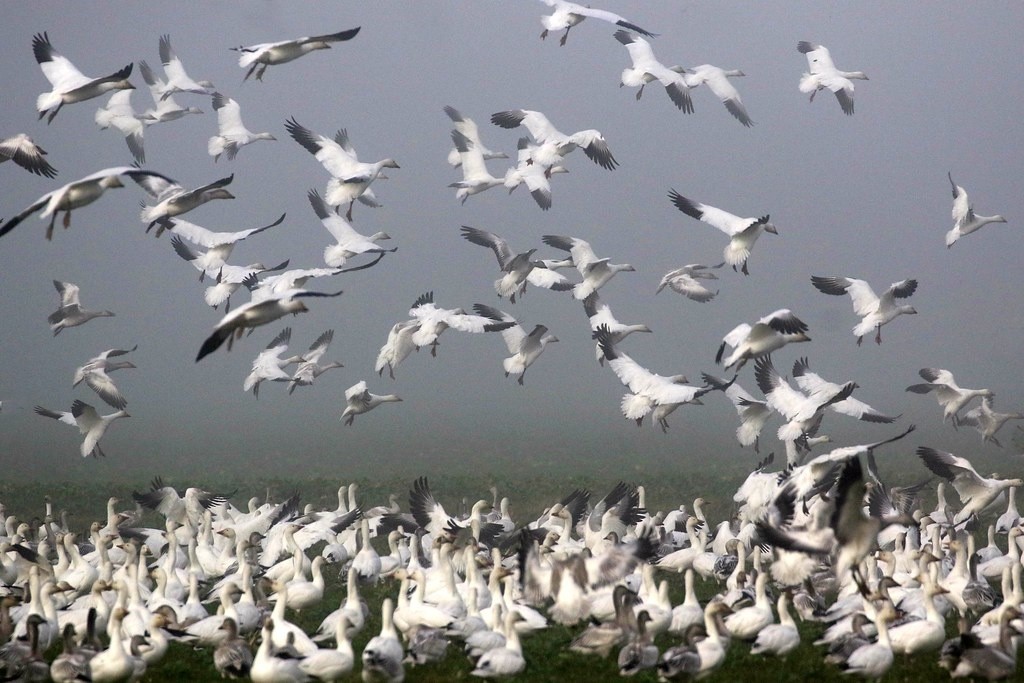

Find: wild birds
<box><xmin>751</xmin><ymin>357</ymin><xmax>903</xmax><ymax>469</ymax></box>
<box><xmin>471</xmin><ymin>304</ymin><xmax>559</xmax><ymax>384</ymax></box>
<box><xmin>0</xmin><ymin>24</ymin><xmax>400</xmax><ymax>456</ymax></box>
<box><xmin>906</xmin><ymin>366</ymin><xmax>995</xmax><ymax>434</ymax></box>
<box><xmin>375</xmin><ymin>292</ymin><xmax>519</xmax><ymax>381</ymax></box>
<box><xmin>0</xmin><ymin>427</ymin><xmax>1024</xmax><ymax>683</ymax></box>
<box><xmin>716</xmin><ymin>308</ymin><xmax>811</xmax><ymax>371</ymax></box>
<box><xmin>809</xmin><ymin>275</ymin><xmax>919</xmax><ymax>347</ymax></box>
<box><xmin>340</xmin><ymin>380</ymin><xmax>402</xmax><ymax>425</ymax></box>
<box><xmin>701</xmin><ymin>372</ymin><xmax>776</xmax><ymax>455</ymax></box>
<box><xmin>943</xmin><ymin>172</ymin><xmax>1006</xmax><ymax>251</ymax></box>
<box><xmin>539</xmin><ymin>0</ymin><xmax>753</xmax><ymax>128</ymax></box>
<box><xmin>797</xmin><ymin>41</ymin><xmax>869</xmax><ymax>114</ymax></box>
<box><xmin>582</xmin><ymin>291</ymin><xmax>653</xmax><ymax>369</ymax></box>
<box><xmin>962</xmin><ymin>395</ymin><xmax>1024</xmax><ymax>448</ymax></box>
<box><xmin>592</xmin><ymin>324</ymin><xmax>710</xmax><ymax>436</ymax></box>
<box><xmin>443</xmin><ymin>104</ymin><xmax>780</xmax><ymax>308</ymax></box>
